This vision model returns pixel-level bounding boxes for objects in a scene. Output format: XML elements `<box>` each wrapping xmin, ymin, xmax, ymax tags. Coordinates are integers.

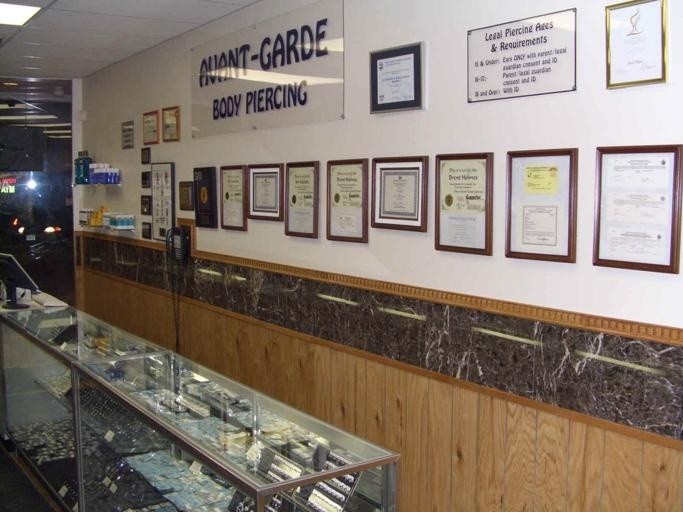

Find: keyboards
<box><xmin>32</xmin><ymin>292</ymin><xmax>68</xmax><ymax>306</ymax></box>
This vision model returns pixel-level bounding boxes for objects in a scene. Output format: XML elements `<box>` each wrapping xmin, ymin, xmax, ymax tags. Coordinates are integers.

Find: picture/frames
<box><xmin>505</xmin><ymin>148</ymin><xmax>579</xmax><ymax>264</ymax></box>
<box><xmin>369</xmin><ymin>41</ymin><xmax>424</xmax><ymax>115</ymax></box>
<box><xmin>604</xmin><ymin>0</ymin><xmax>667</xmax><ymax>90</ymax></box>
<box><xmin>141</xmin><ymin>195</ymin><xmax>152</xmax><ymax>215</ymax></box>
<box><xmin>142</xmin><ymin>110</ymin><xmax>159</xmax><ymax>146</ymax></box>
<box><xmin>220</xmin><ymin>165</ymin><xmax>248</xmax><ymax>232</ymax></box>
<box><xmin>434</xmin><ymin>152</ymin><xmax>494</xmax><ymax>256</ymax></box>
<box><xmin>141</xmin><ymin>222</ymin><xmax>151</xmax><ymax>240</ymax></box>
<box><xmin>177</xmin><ymin>218</ymin><xmax>196</xmax><ymax>250</ymax></box>
<box><xmin>150</xmin><ymin>163</ymin><xmax>175</xmax><ymax>242</ymax></box>
<box><xmin>326</xmin><ymin>158</ymin><xmax>369</xmax><ymax>243</ymax></box>
<box><xmin>162</xmin><ymin>106</ymin><xmax>181</xmax><ymax>142</ymax></box>
<box><xmin>592</xmin><ymin>144</ymin><xmax>683</xmax><ymax>274</ymax></box>
<box><xmin>141</xmin><ymin>147</ymin><xmax>151</xmax><ymax>164</ymax></box>
<box><xmin>248</xmin><ymin>163</ymin><xmax>284</xmax><ymax>221</ymax></box>
<box><xmin>193</xmin><ymin>167</ymin><xmax>218</xmax><ymax>228</ymax></box>
<box><xmin>285</xmin><ymin>161</ymin><xmax>320</xmax><ymax>239</ymax></box>
<box><xmin>178</xmin><ymin>181</ymin><xmax>195</xmax><ymax>211</ymax></box>
<box><xmin>121</xmin><ymin>120</ymin><xmax>134</xmax><ymax>149</ymax></box>
<box><xmin>370</xmin><ymin>156</ymin><xmax>429</xmax><ymax>233</ymax></box>
<box><xmin>141</xmin><ymin>171</ymin><xmax>150</xmax><ymax>188</ymax></box>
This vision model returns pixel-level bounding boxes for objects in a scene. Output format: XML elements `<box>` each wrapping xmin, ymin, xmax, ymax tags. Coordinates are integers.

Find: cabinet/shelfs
<box><xmin>0</xmin><ymin>280</ymin><xmax>401</xmax><ymax>512</ymax></box>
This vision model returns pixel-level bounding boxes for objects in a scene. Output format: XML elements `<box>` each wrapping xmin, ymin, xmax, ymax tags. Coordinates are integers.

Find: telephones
<box><xmin>164</xmin><ymin>225</ymin><xmax>191</xmax><ymax>262</ymax></box>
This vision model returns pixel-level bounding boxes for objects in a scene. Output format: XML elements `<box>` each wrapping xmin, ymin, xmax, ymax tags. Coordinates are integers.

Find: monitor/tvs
<box><xmin>1</xmin><ymin>254</ymin><xmax>39</xmax><ymax>309</ymax></box>
